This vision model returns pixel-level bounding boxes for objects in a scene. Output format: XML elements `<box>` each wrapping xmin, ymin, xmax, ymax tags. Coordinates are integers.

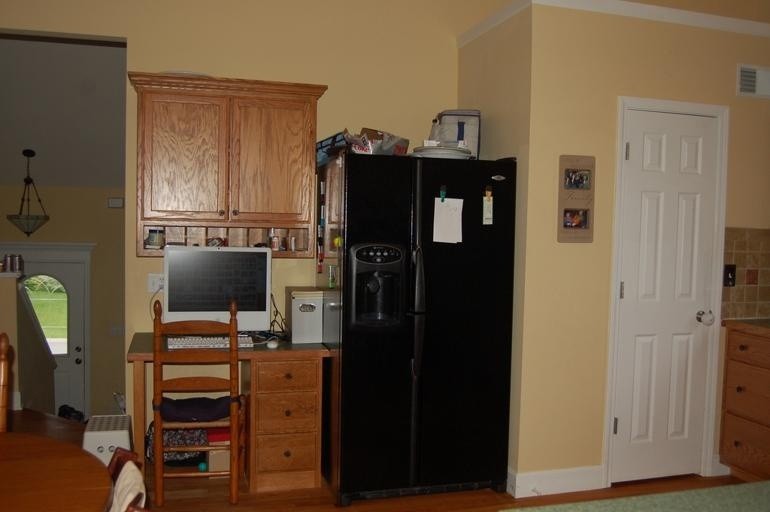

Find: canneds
<box><xmin>290</xmin><ymin>236</ymin><xmax>296</xmax><ymax>250</ymax></box>
<box><xmin>327</xmin><ymin>264</ymin><xmax>336</xmax><ymax>288</ymax></box>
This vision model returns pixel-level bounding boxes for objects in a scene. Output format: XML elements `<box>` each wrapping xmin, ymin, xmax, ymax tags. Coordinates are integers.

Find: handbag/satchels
<box><xmin>145</xmin><ymin>422</ymin><xmax>207</xmax><ymax>466</ymax></box>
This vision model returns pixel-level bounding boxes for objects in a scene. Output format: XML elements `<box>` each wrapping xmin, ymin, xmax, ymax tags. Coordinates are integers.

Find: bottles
<box><xmin>147</xmin><ymin>229</ymin><xmax>165</xmax><ymax>248</ymax></box>
<box><xmin>271</xmin><ymin>237</ymin><xmax>280</xmax><ymax>252</ymax></box>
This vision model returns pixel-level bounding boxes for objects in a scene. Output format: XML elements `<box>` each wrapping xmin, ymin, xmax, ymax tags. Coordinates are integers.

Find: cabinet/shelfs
<box><xmin>126</xmin><ymin>72</ymin><xmax>328</xmax><ymax>258</ymax></box>
<box><xmin>718</xmin><ymin>318</ymin><xmax>769</xmax><ymax>482</ymax></box>
<box><xmin>241</xmin><ymin>359</ymin><xmax>324</xmax><ymax>493</ymax></box>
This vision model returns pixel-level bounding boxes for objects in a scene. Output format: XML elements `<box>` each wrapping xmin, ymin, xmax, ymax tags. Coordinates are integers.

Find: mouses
<box><xmin>267</xmin><ymin>340</ymin><xmax>278</xmax><ymax>349</ymax></box>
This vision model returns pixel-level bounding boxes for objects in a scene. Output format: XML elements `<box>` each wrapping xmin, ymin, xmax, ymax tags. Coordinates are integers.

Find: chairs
<box><xmin>1</xmin><ymin>403</ymin><xmax>150</xmax><ymax>512</ymax></box>
<box><xmin>153</xmin><ymin>297</ymin><xmax>240</xmax><ymax>508</ymax></box>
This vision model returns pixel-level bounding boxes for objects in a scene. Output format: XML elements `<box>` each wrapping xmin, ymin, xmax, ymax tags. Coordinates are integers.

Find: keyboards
<box><xmin>168</xmin><ymin>337</ymin><xmax>254</xmax><ymax>349</ymax></box>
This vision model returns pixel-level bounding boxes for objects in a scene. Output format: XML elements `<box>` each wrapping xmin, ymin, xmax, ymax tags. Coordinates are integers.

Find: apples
<box><xmin>334</xmin><ymin>237</ymin><xmax>344</xmax><ymax>248</ymax></box>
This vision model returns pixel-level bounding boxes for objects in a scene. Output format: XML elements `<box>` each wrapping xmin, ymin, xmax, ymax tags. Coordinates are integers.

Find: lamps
<box><xmin>7</xmin><ymin>150</ymin><xmax>50</xmax><ymax>238</ymax></box>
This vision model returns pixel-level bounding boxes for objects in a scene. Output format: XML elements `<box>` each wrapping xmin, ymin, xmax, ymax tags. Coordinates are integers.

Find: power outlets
<box><xmin>149</xmin><ymin>273</ymin><xmax>165</xmax><ymax>293</ymax></box>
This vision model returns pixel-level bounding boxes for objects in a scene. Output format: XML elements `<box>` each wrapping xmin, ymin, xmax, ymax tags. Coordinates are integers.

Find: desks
<box><xmin>126</xmin><ymin>332</ymin><xmax>332</xmax><ymax>494</ymax></box>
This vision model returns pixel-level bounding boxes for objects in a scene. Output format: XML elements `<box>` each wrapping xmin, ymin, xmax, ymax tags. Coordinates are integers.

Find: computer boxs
<box><xmin>82</xmin><ymin>414</ymin><xmax>132</xmax><ymax>466</ymax></box>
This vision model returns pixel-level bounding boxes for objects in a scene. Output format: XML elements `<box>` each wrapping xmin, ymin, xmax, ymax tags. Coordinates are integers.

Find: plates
<box><xmin>411</xmin><ymin>146</ymin><xmax>473</xmax><ymax>160</ymax></box>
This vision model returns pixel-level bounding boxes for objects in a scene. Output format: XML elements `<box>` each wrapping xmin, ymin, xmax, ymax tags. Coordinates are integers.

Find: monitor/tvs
<box><xmin>163</xmin><ymin>245</ymin><xmax>272</xmax><ymax>332</ymax></box>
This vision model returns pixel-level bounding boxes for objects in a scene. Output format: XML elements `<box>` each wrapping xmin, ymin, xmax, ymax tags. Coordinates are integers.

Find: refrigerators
<box><xmin>321</xmin><ymin>153</ymin><xmax>518</xmax><ymax>507</ymax></box>
